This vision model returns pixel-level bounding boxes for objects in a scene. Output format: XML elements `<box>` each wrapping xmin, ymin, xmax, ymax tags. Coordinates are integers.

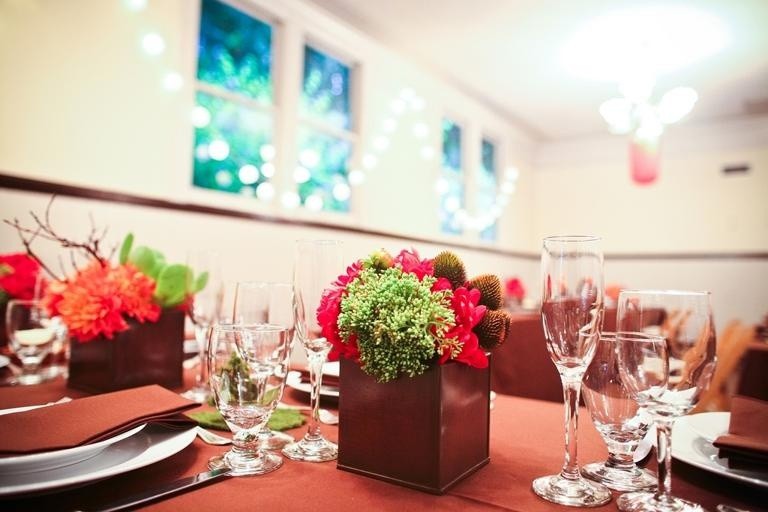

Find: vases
<box><xmin>337</xmin><ymin>353</ymin><xmax>490</xmax><ymax>494</ymax></box>
<box><xmin>64</xmin><ymin>307</ymin><xmax>184</xmax><ymax>396</ymax></box>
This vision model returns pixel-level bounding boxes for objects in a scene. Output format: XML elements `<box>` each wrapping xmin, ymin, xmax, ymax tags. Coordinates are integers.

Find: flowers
<box><xmin>0</xmin><ymin>186</ymin><xmax>209</xmax><ymax>340</ymax></box>
<box><xmin>316</xmin><ymin>245</ymin><xmax>512</xmax><ymax>383</ymax></box>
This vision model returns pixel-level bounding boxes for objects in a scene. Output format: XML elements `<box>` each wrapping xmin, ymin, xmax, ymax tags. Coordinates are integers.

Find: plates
<box><xmin>284</xmin><ymin>360</ymin><xmax>341</xmax><ymax>400</ymax></box>
<box><xmin>0</xmin><ymin>406</ymin><xmax>148</xmax><ymax>477</ymax></box>
<box><xmin>0</xmin><ymin>405</ymin><xmax>201</xmax><ymax>497</ymax></box>
<box><xmin>670</xmin><ymin>412</ymin><xmax>767</xmax><ymax>485</ymax></box>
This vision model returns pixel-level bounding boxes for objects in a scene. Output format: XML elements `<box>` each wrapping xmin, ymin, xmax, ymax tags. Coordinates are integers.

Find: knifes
<box><xmin>62</xmin><ymin>468</ymin><xmax>234</xmax><ymax>512</ymax></box>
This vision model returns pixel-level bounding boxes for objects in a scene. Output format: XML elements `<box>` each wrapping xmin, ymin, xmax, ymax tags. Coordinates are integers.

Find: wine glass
<box><xmin>582</xmin><ymin>330</ymin><xmax>671</xmax><ymax>492</ymax></box>
<box><xmin>281</xmin><ymin>238</ymin><xmax>346</xmax><ymax>466</ymax></box>
<box><xmin>530</xmin><ymin>232</ymin><xmax>614</xmax><ymax>508</ymax></box>
<box><xmin>613</xmin><ymin>289</ymin><xmax>718</xmax><ymax>512</ymax></box>
<box><xmin>181</xmin><ymin>259</ymin><xmax>227</xmax><ymax>403</ymax></box>
<box><xmin>232</xmin><ymin>279</ymin><xmax>298</xmax><ymax>450</ymax></box>
<box><xmin>207</xmin><ymin>324</ymin><xmax>287</xmax><ymax>476</ymax></box>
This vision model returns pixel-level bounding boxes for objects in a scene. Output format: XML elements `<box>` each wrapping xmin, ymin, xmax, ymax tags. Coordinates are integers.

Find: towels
<box><xmin>714</xmin><ymin>396</ymin><xmax>768</xmax><ymax>474</ymax></box>
<box><xmin>0</xmin><ymin>384</ymin><xmax>201</xmax><ymax>455</ymax></box>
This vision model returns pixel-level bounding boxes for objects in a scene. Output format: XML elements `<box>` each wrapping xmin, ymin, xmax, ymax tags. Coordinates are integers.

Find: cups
<box><xmin>6</xmin><ymin>299</ymin><xmax>62</xmax><ymax>385</ymax></box>
